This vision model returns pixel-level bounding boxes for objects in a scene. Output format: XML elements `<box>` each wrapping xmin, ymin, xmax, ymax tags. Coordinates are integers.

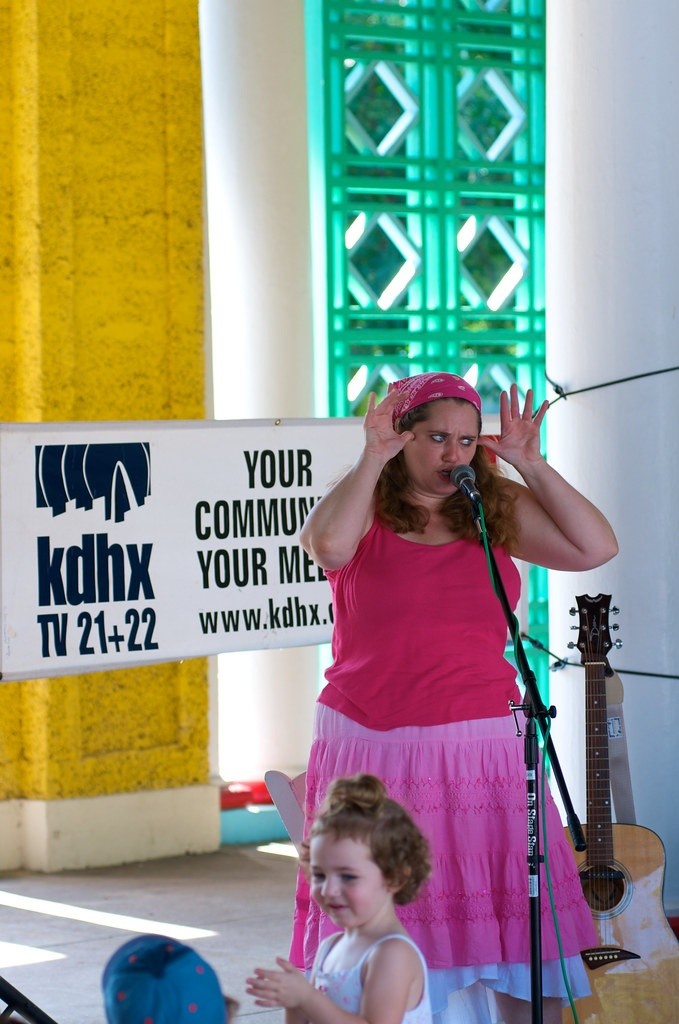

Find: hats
<box><xmin>101</xmin><ymin>935</ymin><xmax>229</xmax><ymax>1024</ymax></box>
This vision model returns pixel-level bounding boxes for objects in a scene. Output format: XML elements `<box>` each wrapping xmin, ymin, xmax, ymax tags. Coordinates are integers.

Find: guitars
<box><xmin>559</xmin><ymin>593</ymin><xmax>679</xmax><ymax>1023</ymax></box>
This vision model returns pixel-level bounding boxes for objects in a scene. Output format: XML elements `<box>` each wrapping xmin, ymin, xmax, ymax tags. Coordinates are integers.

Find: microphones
<box><xmin>451</xmin><ymin>464</ymin><xmax>481</xmax><ymax>502</ymax></box>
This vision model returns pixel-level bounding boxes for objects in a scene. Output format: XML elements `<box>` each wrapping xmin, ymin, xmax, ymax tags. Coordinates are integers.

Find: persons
<box><xmin>101</xmin><ymin>935</ymin><xmax>240</xmax><ymax>1024</ymax></box>
<box><xmin>288</xmin><ymin>372</ymin><xmax>619</xmax><ymax>1024</ymax></box>
<box><xmin>246</xmin><ymin>774</ymin><xmax>433</xmax><ymax>1024</ymax></box>
